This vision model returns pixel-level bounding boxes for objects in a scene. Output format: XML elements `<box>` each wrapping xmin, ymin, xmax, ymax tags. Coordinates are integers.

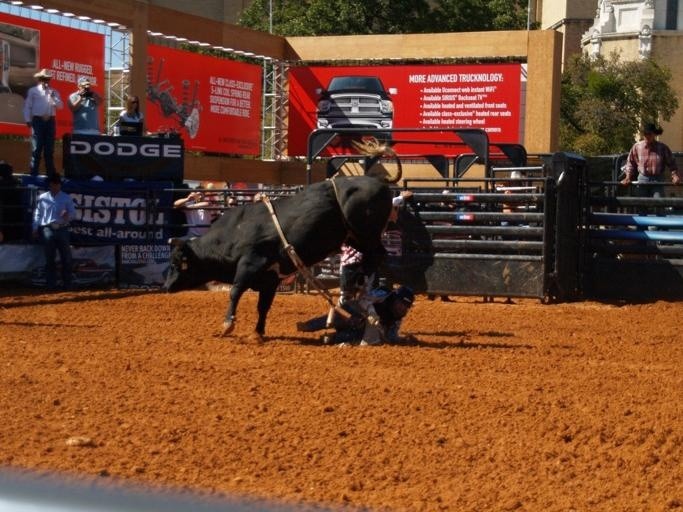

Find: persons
<box><xmin>619</xmin><ymin>123</ymin><xmax>680</xmax><ymax>250</ymax></box>
<box><xmin>173</xmin><ymin>185</ymin><xmax>212</xmax><ymax>238</ymax></box>
<box><xmin>66</xmin><ymin>75</ymin><xmax>103</xmax><ymax>136</ymax></box>
<box><xmin>294</xmin><ymin>285</ymin><xmax>416</xmax><ymax>346</ymax></box>
<box><xmin>30</xmin><ymin>173</ymin><xmax>77</xmax><ymax>293</ymax></box>
<box><xmin>112</xmin><ymin>96</ymin><xmax>152</xmax><ymax>137</ymax></box>
<box><xmin>22</xmin><ymin>69</ymin><xmax>64</xmax><ymax>176</ymax></box>
<box><xmin>223</xmin><ymin>196</ymin><xmax>236</xmax><ymax>209</ymax></box>
<box><xmin>315</xmin><ymin>186</ymin><xmax>539</xmax><ymax>306</ymax></box>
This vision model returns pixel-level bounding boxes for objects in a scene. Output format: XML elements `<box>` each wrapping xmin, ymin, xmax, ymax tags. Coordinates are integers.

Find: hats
<box><xmin>34</xmin><ymin>68</ymin><xmax>54</xmax><ymax>78</ymax></box>
<box><xmin>77</xmin><ymin>76</ymin><xmax>90</xmax><ymax>85</ymax></box>
<box><xmin>44</xmin><ymin>172</ymin><xmax>63</xmax><ymax>183</ymax></box>
<box><xmin>394</xmin><ymin>285</ymin><xmax>415</xmax><ymax>308</ymax></box>
<box><xmin>639</xmin><ymin>122</ymin><xmax>662</xmax><ymax>135</ymax></box>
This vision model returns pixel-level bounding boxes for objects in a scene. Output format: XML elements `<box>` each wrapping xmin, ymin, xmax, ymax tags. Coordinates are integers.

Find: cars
<box><xmin>313</xmin><ymin>73</ymin><xmax>398</xmax><ymax>140</ymax></box>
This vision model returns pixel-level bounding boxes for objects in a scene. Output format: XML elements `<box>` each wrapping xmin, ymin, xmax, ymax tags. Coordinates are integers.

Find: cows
<box><xmin>162</xmin><ymin>135</ymin><xmax>403</xmax><ymax>344</ymax></box>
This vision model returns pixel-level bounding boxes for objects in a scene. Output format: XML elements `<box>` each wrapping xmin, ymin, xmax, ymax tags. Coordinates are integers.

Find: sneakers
<box><xmin>296</xmin><ymin>322</ymin><xmax>310</xmax><ymax>332</ymax></box>
